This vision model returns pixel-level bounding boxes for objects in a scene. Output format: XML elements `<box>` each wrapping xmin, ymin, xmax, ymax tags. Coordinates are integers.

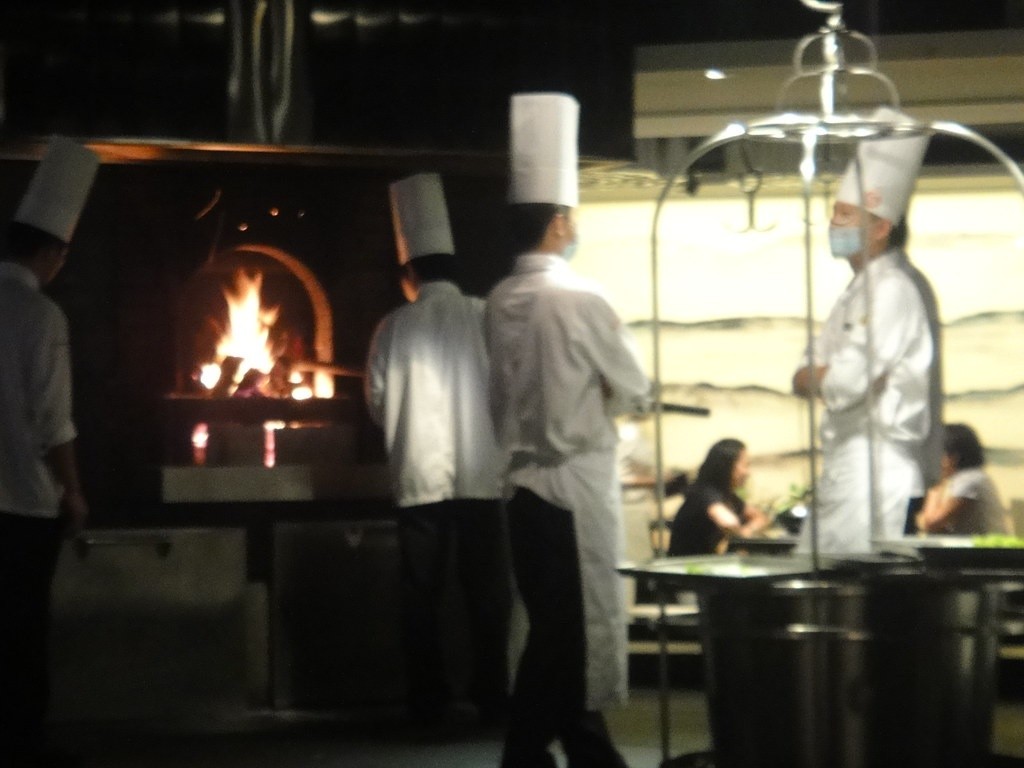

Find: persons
<box><xmin>666</xmin><ymin>438</ymin><xmax>769</xmax><ymax>556</ymax></box>
<box><xmin>363</xmin><ymin>173</ymin><xmax>512</xmax><ymax>743</ymax></box>
<box><xmin>0</xmin><ymin>139</ymin><xmax>100</xmax><ymax>721</ymax></box>
<box><xmin>792</xmin><ymin>106</ymin><xmax>934</xmax><ymax>555</ymax></box>
<box><xmin>914</xmin><ymin>424</ymin><xmax>1012</xmax><ymax>535</ymax></box>
<box><xmin>480</xmin><ymin>94</ymin><xmax>654</xmax><ymax>768</ymax></box>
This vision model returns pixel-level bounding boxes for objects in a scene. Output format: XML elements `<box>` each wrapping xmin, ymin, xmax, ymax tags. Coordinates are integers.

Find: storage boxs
<box><xmin>50</xmin><ymin>528</ymin><xmax>247</xmax><ymax>616</ymax></box>
<box><xmin>51</xmin><ymin>583</ymin><xmax>271</xmax><ymax>715</ymax></box>
